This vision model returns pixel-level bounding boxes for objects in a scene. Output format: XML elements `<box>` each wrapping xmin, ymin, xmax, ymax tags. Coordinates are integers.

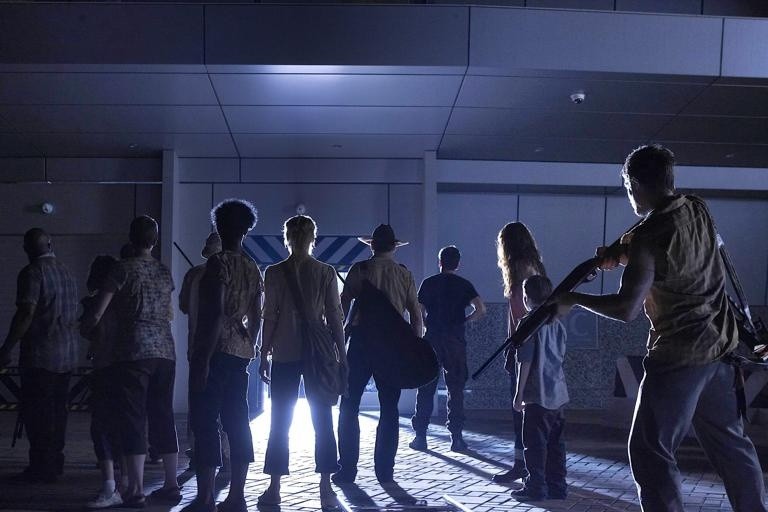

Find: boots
<box><xmin>450</xmin><ymin>436</ymin><xmax>467</xmax><ymax>451</ymax></box>
<box><xmin>409</xmin><ymin>431</ymin><xmax>427</xmax><ymax>449</ymax></box>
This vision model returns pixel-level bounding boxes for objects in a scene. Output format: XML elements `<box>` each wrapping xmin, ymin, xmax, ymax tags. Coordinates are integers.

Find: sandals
<box><xmin>150</xmin><ymin>486</ymin><xmax>183</xmax><ymax>501</ymax></box>
<box><xmin>122</xmin><ymin>492</ymin><xmax>147</xmax><ymax>508</ymax></box>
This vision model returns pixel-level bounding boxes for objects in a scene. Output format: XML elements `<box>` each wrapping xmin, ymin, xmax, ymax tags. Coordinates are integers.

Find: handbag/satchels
<box><xmin>301</xmin><ymin>324</ymin><xmax>344</xmax><ymax>406</ymax></box>
<box><xmin>358</xmin><ymin>280</ymin><xmax>440</xmax><ymax>390</ymax></box>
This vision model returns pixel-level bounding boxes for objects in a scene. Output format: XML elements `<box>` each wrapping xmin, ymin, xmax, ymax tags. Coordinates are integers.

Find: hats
<box><xmin>202</xmin><ymin>232</ymin><xmax>222</xmax><ymax>258</ymax></box>
<box><xmin>357</xmin><ymin>224</ymin><xmax>409</xmax><ymax>247</ymax></box>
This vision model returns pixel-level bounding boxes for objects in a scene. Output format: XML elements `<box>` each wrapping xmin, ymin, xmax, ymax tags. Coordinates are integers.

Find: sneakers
<box><xmin>217</xmin><ymin>500</ymin><xmax>247</xmax><ymax>511</ymax></box>
<box><xmin>84</xmin><ymin>489</ymin><xmax>123</xmax><ymax>509</ymax></box>
<box><xmin>179</xmin><ymin>502</ymin><xmax>215</xmax><ymax>512</ymax></box>
<box><xmin>330</xmin><ymin>469</ymin><xmax>356</xmax><ymax>483</ymax></box>
<box><xmin>258</xmin><ymin>489</ymin><xmax>282</xmax><ymax>505</ymax></box>
<box><xmin>511</xmin><ymin>489</ymin><xmax>544</xmax><ymax>501</ymax></box>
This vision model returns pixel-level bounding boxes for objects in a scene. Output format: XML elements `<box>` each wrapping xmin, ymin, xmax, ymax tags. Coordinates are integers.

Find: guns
<box><xmin>471</xmin><ymin>219</ymin><xmax>644</xmax><ymax>379</ymax></box>
<box><xmin>1</xmin><ymin>371</ymin><xmax>23</xmax><ymax>447</ymax></box>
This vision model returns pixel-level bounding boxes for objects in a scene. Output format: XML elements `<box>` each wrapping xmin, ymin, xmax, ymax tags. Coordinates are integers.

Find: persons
<box><xmin>489</xmin><ymin>221</ymin><xmax>554</xmax><ymax>487</ymax></box>
<box><xmin>80</xmin><ymin>209</ymin><xmax>186</xmax><ymax>506</ymax></box>
<box><xmin>1</xmin><ymin>226</ymin><xmax>81</xmax><ymax>487</ymax></box>
<box><xmin>407</xmin><ymin>244</ymin><xmax>470</xmax><ymax>454</ymax></box>
<box><xmin>178</xmin><ymin>229</ymin><xmax>260</xmax><ymax>486</ymax></box>
<box><xmin>329</xmin><ymin>222</ymin><xmax>423</xmax><ymax>486</ymax></box>
<box><xmin>544</xmin><ymin>137</ymin><xmax>767</xmax><ymax>510</ymax></box>
<box><xmin>507</xmin><ymin>272</ymin><xmax>572</xmax><ymax>503</ymax></box>
<box><xmin>254</xmin><ymin>213</ymin><xmax>354</xmax><ymax>508</ymax></box>
<box><xmin>174</xmin><ymin>198</ymin><xmax>266</xmax><ymax>512</ymax></box>
<box><xmin>78</xmin><ymin>251</ymin><xmax>132</xmax><ymax>509</ymax></box>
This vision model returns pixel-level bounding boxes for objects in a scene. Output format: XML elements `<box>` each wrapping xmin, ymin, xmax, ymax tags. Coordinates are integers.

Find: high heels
<box><xmin>492</xmin><ymin>464</ymin><xmax>528</xmax><ymax>483</ymax></box>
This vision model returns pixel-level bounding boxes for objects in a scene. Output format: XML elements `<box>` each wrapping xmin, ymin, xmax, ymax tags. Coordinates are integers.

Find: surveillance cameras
<box><xmin>42</xmin><ymin>203</ymin><xmax>53</xmax><ymax>215</ymax></box>
<box><xmin>570</xmin><ymin>94</ymin><xmax>585</xmax><ymax>104</ymax></box>
